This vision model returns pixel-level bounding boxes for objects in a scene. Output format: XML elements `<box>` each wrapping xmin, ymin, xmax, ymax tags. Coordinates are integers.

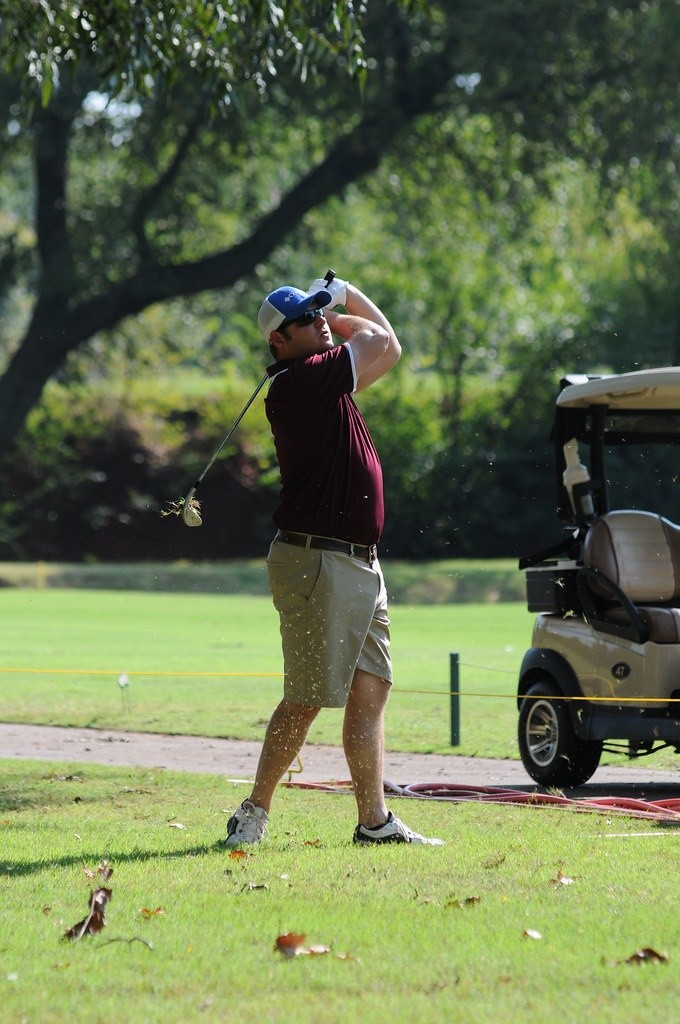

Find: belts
<box><xmin>275</xmin><ymin>528</ymin><xmax>378</xmax><ymax>563</ymax></box>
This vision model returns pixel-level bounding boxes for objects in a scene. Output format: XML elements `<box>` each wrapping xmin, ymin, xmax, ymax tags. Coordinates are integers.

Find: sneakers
<box><xmin>223</xmin><ymin>798</ymin><xmax>270</xmax><ymax>847</ymax></box>
<box><xmin>353</xmin><ymin>811</ymin><xmax>446</xmax><ymax>847</ymax></box>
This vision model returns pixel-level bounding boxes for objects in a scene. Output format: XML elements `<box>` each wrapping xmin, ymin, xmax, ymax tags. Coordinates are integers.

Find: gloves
<box><xmin>306</xmin><ymin>278</ymin><xmax>349</xmax><ymax>310</ymax></box>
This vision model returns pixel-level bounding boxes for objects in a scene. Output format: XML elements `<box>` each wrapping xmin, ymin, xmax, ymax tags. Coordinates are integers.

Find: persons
<box><xmin>226</xmin><ymin>277</ymin><xmax>442</xmax><ymax>848</ymax></box>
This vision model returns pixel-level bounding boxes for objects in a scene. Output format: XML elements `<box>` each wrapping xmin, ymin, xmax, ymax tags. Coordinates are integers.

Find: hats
<box><xmin>258</xmin><ymin>285</ymin><xmax>333</xmax><ymax>345</ymax></box>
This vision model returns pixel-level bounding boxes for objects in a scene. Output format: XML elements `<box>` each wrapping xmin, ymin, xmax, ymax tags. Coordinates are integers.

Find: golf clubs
<box><xmin>181</xmin><ymin>268</ymin><xmax>336</xmax><ymax>528</ymax></box>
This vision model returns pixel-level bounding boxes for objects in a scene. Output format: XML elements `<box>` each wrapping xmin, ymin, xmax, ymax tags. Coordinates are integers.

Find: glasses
<box><xmin>269</xmin><ymin>306</ymin><xmax>324</xmax><ymax>345</ymax></box>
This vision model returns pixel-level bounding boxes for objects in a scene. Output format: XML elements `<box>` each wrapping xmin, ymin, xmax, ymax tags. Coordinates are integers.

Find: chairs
<box><xmin>584</xmin><ymin>510</ymin><xmax>680</xmax><ymax>644</ymax></box>
